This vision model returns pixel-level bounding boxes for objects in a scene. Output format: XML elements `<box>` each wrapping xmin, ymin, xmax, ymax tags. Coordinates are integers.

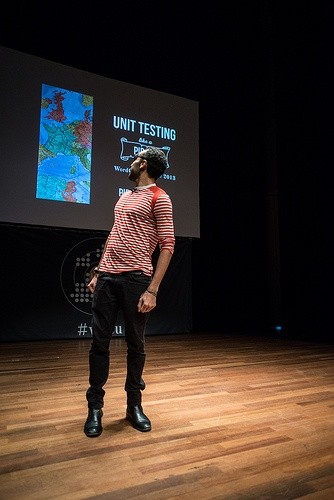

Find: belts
<box><xmin>97</xmin><ymin>266</ymin><xmax>145</xmax><ymax>277</ymax></box>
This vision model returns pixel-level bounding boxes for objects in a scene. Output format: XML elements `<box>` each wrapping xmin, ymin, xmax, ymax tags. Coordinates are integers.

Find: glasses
<box><xmin>132</xmin><ymin>154</ymin><xmax>150</xmax><ymax>162</ymax></box>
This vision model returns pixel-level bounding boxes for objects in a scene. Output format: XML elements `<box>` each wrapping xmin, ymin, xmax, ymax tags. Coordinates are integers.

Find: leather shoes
<box><xmin>84</xmin><ymin>406</ymin><xmax>104</xmax><ymax>437</ymax></box>
<box><xmin>126</xmin><ymin>402</ymin><xmax>151</xmax><ymax>432</ymax></box>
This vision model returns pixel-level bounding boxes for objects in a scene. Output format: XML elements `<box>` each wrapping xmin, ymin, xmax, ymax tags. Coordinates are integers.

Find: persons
<box><xmin>81</xmin><ymin>145</ymin><xmax>175</xmax><ymax>438</ymax></box>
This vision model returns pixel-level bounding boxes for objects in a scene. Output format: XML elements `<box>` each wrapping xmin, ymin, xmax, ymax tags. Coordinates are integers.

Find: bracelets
<box><xmin>146</xmin><ymin>288</ymin><xmax>159</xmax><ymax>298</ymax></box>
